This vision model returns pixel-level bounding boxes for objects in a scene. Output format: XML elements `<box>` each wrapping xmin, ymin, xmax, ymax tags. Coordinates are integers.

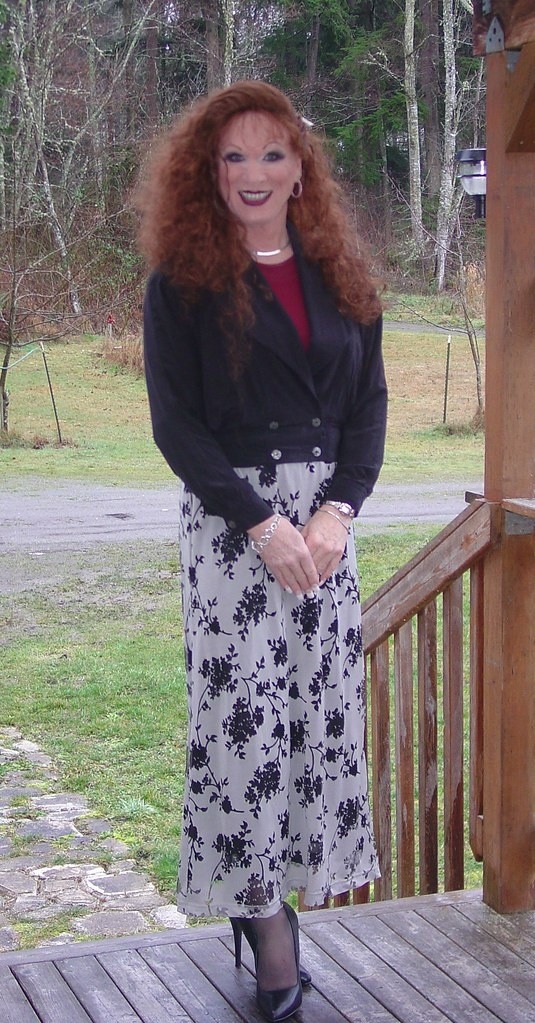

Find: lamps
<box><xmin>455</xmin><ymin>149</ymin><xmax>487</xmax><ymax>221</ymax></box>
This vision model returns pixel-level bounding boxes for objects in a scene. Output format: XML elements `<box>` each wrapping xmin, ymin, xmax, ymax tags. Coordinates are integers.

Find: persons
<box><xmin>128</xmin><ymin>78</ymin><xmax>389</xmax><ymax>1023</ymax></box>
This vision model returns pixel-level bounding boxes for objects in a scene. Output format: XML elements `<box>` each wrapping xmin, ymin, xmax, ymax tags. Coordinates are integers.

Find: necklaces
<box><xmin>250</xmin><ymin>239</ymin><xmax>291</xmax><ymax>257</ymax></box>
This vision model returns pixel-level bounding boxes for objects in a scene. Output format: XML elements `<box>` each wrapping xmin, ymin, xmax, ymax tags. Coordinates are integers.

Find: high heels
<box><xmin>244</xmin><ymin>900</ymin><xmax>304</xmax><ymax>1022</ymax></box>
<box><xmin>228</xmin><ymin>917</ymin><xmax>312</xmax><ymax>986</ymax></box>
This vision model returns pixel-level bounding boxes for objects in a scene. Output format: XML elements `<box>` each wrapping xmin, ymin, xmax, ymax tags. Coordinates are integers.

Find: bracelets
<box><xmin>326</xmin><ymin>500</ymin><xmax>356</xmax><ymax>517</ymax></box>
<box><xmin>318</xmin><ymin>508</ymin><xmax>351</xmax><ymax>537</ymax></box>
<box><xmin>251</xmin><ymin>513</ymin><xmax>281</xmax><ymax>554</ymax></box>
